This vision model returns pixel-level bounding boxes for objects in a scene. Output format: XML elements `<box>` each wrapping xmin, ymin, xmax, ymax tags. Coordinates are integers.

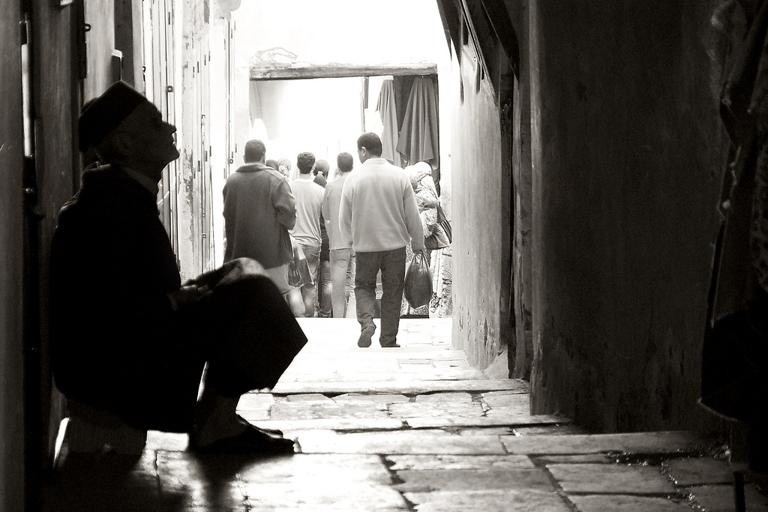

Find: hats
<box><xmin>76</xmin><ymin>79</ymin><xmax>147</xmax><ymax>154</ymax></box>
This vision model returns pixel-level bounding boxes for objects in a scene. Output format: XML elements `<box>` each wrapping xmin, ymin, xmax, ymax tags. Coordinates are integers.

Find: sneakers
<box><xmin>356</xmin><ymin>326</ymin><xmax>401</xmax><ymax>349</ymax></box>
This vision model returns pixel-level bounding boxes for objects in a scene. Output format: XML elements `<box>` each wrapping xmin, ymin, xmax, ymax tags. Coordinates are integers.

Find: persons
<box><xmin>51</xmin><ymin>82</ymin><xmax>308</xmax><ymax>460</ymax></box>
<box><xmin>339</xmin><ymin>131</ymin><xmax>424</xmax><ymax>347</ymax></box>
<box><xmin>224</xmin><ymin>139</ymin><xmax>440</xmax><ymax>317</ymax></box>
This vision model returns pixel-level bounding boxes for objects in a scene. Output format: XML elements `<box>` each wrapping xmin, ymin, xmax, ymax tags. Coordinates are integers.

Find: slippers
<box><xmin>185</xmin><ymin>414</ymin><xmax>295</xmax><ymax>456</ymax></box>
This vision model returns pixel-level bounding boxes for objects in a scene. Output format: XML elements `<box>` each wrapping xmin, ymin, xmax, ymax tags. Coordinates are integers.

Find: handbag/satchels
<box><xmin>403</xmin><ymin>250</ymin><xmax>434</xmax><ymax>310</ymax></box>
<box><xmin>288</xmin><ymin>248</ymin><xmax>313</xmax><ymax>290</ymax></box>
